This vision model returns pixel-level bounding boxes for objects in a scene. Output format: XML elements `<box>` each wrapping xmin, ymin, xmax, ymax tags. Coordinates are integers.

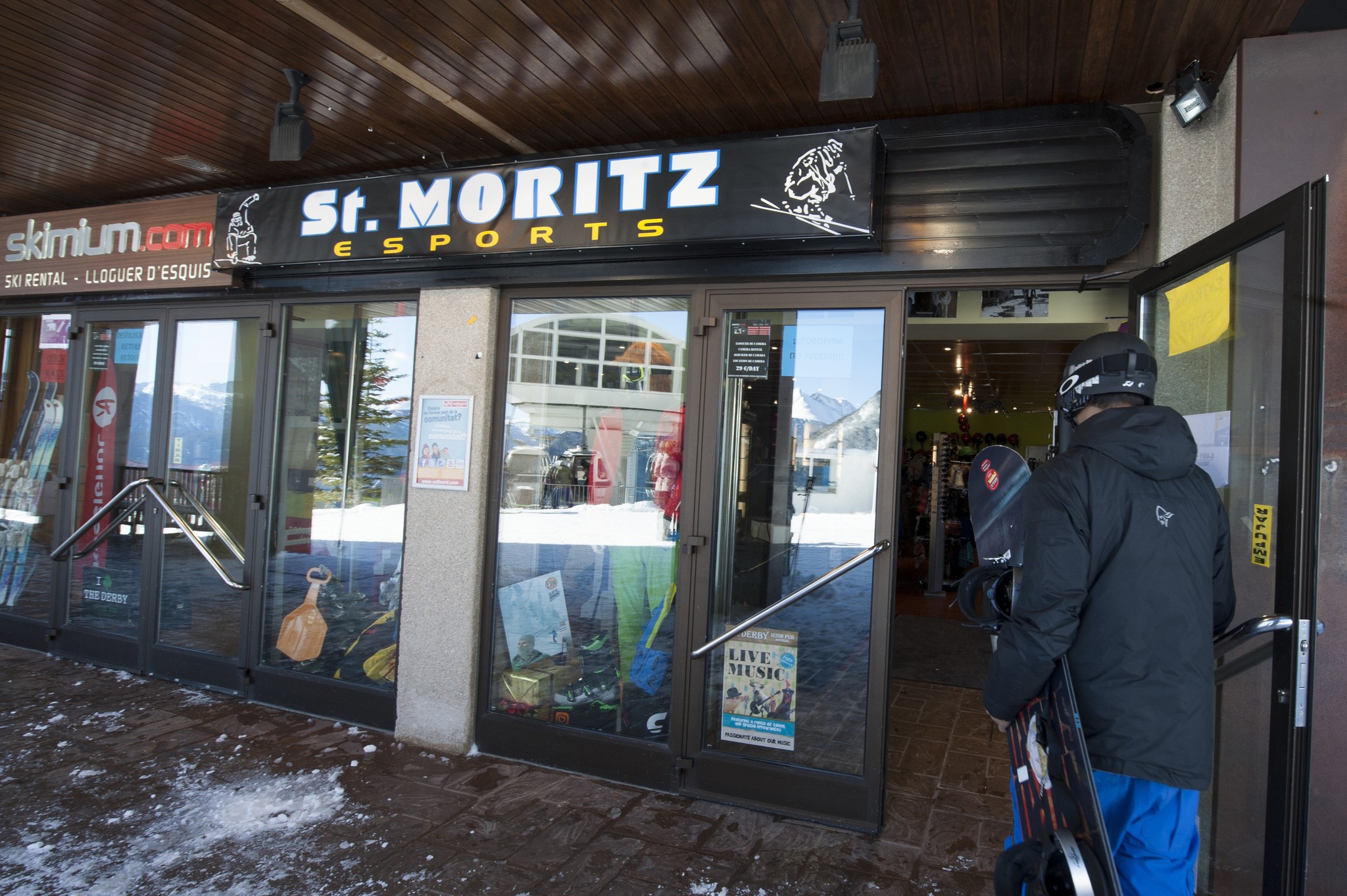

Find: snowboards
<box><xmin>958</xmin><ymin>443</ymin><xmax>1121</xmax><ymax>896</ymax></box>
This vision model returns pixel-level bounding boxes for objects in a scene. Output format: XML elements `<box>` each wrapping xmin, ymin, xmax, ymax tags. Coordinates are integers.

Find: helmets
<box><xmin>1007</xmin><ymin>432</ymin><xmax>1020</xmax><ymax>448</ymax></box>
<box><xmin>962</xmin><ymin>434</ymin><xmax>971</xmax><ymax>445</ymax></box>
<box><xmin>984</xmin><ymin>432</ymin><xmax>996</xmax><ymax>447</ymax></box>
<box><xmin>959</xmin><ymin>421</ymin><xmax>971</xmax><ymax>432</ymax></box>
<box><xmin>996</xmin><ymin>432</ymin><xmax>1006</xmax><ymax>447</ymax></box>
<box><xmin>948</xmin><ymin>432</ymin><xmax>959</xmax><ymax>443</ymax></box>
<box><xmin>956</xmin><ymin>414</ymin><xmax>968</xmax><ymax>424</ymax></box>
<box><xmin>1056</xmin><ymin>332</ymin><xmax>1158</xmax><ymax>418</ymax></box>
<box><xmin>917</xmin><ymin>431</ymin><xmax>928</xmax><ymax>443</ymax></box>
<box><xmin>958</xmin><ymin>447</ymin><xmax>977</xmax><ymax>464</ymax></box>
<box><xmin>971</xmin><ymin>432</ymin><xmax>983</xmax><ymax>448</ymax></box>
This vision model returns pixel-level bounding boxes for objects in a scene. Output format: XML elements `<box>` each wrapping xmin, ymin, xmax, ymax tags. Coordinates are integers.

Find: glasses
<box><xmin>619</xmin><ymin>365</ymin><xmax>672</xmax><ymax>382</ymax></box>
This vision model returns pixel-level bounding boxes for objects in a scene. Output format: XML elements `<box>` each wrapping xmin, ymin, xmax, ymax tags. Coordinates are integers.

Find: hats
<box><xmin>726</xmin><ymin>688</ymin><xmax>742</xmax><ymax>699</ymax></box>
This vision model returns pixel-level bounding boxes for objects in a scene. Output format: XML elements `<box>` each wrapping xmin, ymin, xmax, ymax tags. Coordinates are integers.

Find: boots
<box><xmin>551</xmin><ymin>630</ymin><xmax>621</xmax><ymax>709</ymax></box>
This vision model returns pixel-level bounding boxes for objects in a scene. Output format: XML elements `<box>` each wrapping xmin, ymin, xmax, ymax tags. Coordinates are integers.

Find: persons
<box><xmin>419</xmin><ymin>443</ymin><xmax>452</xmax><ymax>464</ymax></box>
<box><xmin>510</xmin><ymin>634</ymin><xmax>551</xmax><ymax>670</ymax></box>
<box><xmin>545</xmin><ymin>459</ymin><xmax>590</xmax><ymax>510</ymax></box>
<box><xmin>653</xmin><ymin>440</ymin><xmax>682</xmax><ymax>539</ymax></box>
<box><xmin>549</xmin><ymin>628</ymin><xmax>558</xmax><ymax>643</ymax></box>
<box><xmin>974</xmin><ymin>332</ymin><xmax>1240</xmax><ymax>896</ymax></box>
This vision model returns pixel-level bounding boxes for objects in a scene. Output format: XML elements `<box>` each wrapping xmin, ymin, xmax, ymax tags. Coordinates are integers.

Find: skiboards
<box><xmin>0</xmin><ymin>370</ymin><xmax>59</xmax><ymax>573</ymax></box>
<box><xmin>0</xmin><ymin>400</ymin><xmax>64</xmax><ymax>606</ymax></box>
